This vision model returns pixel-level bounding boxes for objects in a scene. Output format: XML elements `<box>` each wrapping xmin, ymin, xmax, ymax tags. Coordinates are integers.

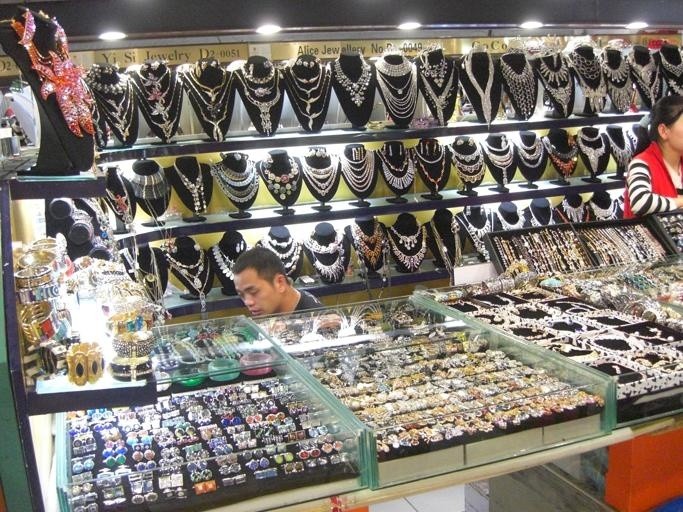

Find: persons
<box><xmin>620</xmin><ymin>93</ymin><xmax>682</xmax><ymax>218</ymax></box>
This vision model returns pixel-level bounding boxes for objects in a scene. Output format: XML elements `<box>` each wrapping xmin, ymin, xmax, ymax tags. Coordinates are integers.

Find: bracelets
<box><xmin>109</xmin><ymin>356</ymin><xmax>154</xmax><ymax>381</ymax></box>
<box><xmin>65</xmin><ymin>341</ymin><xmax>105</xmax><ymax>386</ymax></box>
<box><xmin>150</xmin><ymin>323</ymin><xmax>281</xmax><ymax>371</ymax></box>
<box><xmin>11</xmin><ymin>237</ymin><xmax>159</xmax><ymax>370</ymax></box>
<box><xmin>23</xmin><ymin>337</ymin><xmax>66</xmax><ymax>382</ymax></box>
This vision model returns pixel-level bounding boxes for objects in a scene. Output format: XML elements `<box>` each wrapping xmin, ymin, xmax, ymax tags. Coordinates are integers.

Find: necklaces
<box><xmin>258</xmin><ymin>282</ymin><xmax>683</xmax><ymax>372</ymax></box>
<box><xmin>10</xmin><ymin>8</ymin><xmax>682</xmax><ymax>144</ymax></box>
<box><xmin>64</xmin><ymin>124</ymin><xmax>681</xmax><ymax>318</ymax></box>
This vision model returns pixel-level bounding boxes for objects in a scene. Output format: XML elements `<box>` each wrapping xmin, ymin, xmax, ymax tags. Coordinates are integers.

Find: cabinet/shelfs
<box><xmin>2</xmin><ymin>45</ymin><xmax>676</xmax><ymax>422</ymax></box>
